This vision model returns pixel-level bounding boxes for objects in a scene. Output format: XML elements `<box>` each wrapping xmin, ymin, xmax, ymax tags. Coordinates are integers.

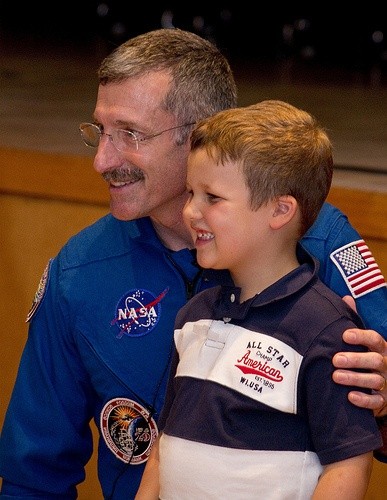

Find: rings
<box><xmin>374</xmin><ymin>373</ymin><xmax>387</xmax><ymax>391</ymax></box>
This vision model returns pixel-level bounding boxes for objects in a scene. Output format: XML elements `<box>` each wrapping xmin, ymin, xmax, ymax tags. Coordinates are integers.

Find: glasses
<box><xmin>78</xmin><ymin>121</ymin><xmax>196</xmax><ymax>154</ymax></box>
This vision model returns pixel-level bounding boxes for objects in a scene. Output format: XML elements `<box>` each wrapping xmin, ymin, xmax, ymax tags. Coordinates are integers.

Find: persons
<box><xmin>1</xmin><ymin>29</ymin><xmax>387</xmax><ymax>500</ymax></box>
<box><xmin>133</xmin><ymin>100</ymin><xmax>384</xmax><ymax>499</ymax></box>
